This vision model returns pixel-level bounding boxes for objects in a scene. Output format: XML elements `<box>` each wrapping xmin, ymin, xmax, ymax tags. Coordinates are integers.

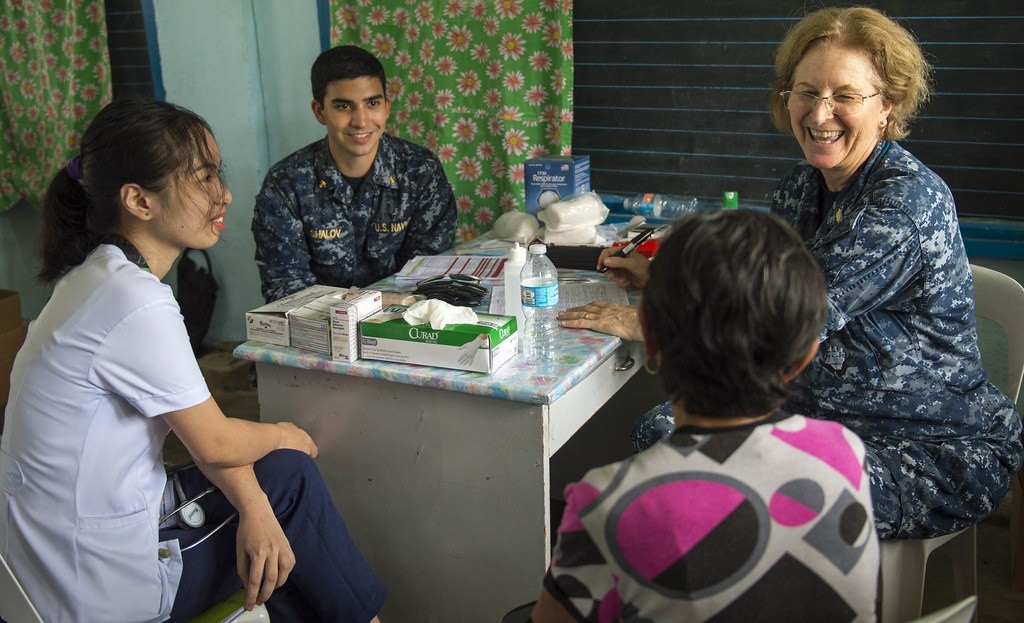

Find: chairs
<box><xmin>0</xmin><ymin>549</ymin><xmax>273</xmax><ymax>623</ymax></box>
<box><xmin>862</xmin><ymin>265</ymin><xmax>1024</xmax><ymax>623</ymax></box>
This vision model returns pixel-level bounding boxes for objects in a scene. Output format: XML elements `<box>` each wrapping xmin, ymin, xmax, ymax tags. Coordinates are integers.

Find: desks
<box><xmin>232</xmin><ymin>192</ymin><xmax>664</xmax><ymax>623</ymax></box>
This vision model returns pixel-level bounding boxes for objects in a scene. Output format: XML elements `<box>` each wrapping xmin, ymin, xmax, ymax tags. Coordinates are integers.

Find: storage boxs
<box><xmin>523</xmin><ymin>154</ymin><xmax>591</xmax><ymax>229</ymax></box>
<box><xmin>0</xmin><ymin>290</ymin><xmax>23</xmax><ymax>334</ymax></box>
<box><xmin>246</xmin><ymin>283</ymin><xmax>518</xmax><ymax>375</ymax></box>
<box><xmin>0</xmin><ymin>321</ymin><xmax>26</xmax><ymax>409</ymax></box>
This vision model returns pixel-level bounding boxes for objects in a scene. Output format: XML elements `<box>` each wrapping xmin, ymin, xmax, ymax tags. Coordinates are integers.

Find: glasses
<box><xmin>779</xmin><ymin>90</ymin><xmax>880</xmax><ymax>115</ymax></box>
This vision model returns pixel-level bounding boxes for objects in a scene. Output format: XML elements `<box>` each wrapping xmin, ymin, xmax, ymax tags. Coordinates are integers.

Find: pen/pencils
<box><xmin>595</xmin><ymin>227</ymin><xmax>656</xmax><ymax>273</ymax></box>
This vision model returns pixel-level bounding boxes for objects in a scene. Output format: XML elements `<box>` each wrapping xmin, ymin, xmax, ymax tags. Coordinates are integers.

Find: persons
<box><xmin>0</xmin><ymin>98</ymin><xmax>387</xmax><ymax>623</ymax></box>
<box><xmin>557</xmin><ymin>8</ymin><xmax>1024</xmax><ymax>542</ymax></box>
<box><xmin>534</xmin><ymin>207</ymin><xmax>881</xmax><ymax>623</ymax></box>
<box><xmin>251</xmin><ymin>45</ymin><xmax>457</xmax><ymax>306</ymax></box>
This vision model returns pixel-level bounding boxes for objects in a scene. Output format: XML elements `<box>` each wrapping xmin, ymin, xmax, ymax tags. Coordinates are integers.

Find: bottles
<box><xmin>503</xmin><ymin>245</ymin><xmax>529</xmax><ymax>317</ymax></box>
<box><xmin>623</xmin><ymin>193</ymin><xmax>698</xmax><ymax>220</ymax></box>
<box><xmin>519</xmin><ymin>245</ymin><xmax>560</xmax><ymax>364</ymax></box>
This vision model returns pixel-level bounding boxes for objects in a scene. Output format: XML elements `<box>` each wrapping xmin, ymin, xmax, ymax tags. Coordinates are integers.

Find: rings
<box><xmin>585</xmin><ymin>312</ymin><xmax>589</xmax><ymax>319</ymax></box>
